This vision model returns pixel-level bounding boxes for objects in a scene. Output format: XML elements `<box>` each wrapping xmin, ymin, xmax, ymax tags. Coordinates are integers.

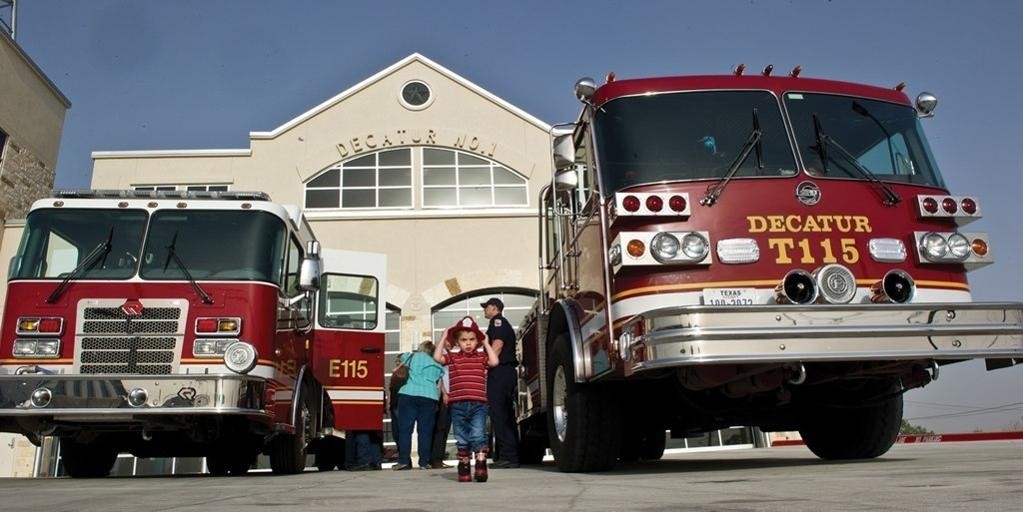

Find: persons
<box><xmin>432</xmin><ymin>316</ymin><xmax>499</xmax><ymax>483</ymax></box>
<box><xmin>479</xmin><ymin>297</ymin><xmax>520</xmax><ymax>469</ymax></box>
<box><xmin>341</xmin><ymin>339</ymin><xmax>452</xmax><ymax>470</ymax></box>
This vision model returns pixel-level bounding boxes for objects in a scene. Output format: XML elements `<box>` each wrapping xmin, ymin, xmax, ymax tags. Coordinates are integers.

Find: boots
<box><xmin>474</xmin><ymin>448</ymin><xmax>489</xmax><ymax>481</ymax></box>
<box><xmin>456</xmin><ymin>451</ymin><xmax>471</xmax><ymax>481</ymax></box>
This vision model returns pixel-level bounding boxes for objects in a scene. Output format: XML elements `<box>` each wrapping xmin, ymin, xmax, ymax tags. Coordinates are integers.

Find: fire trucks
<box><xmin>1</xmin><ymin>185</ymin><xmax>391</xmax><ymax>477</ymax></box>
<box><xmin>480</xmin><ymin>61</ymin><xmax>1023</xmax><ymax>472</ymax></box>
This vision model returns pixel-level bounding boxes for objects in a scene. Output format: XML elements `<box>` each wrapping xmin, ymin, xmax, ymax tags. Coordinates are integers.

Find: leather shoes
<box><xmin>488</xmin><ymin>459</ymin><xmax>521</xmax><ymax>469</ymax></box>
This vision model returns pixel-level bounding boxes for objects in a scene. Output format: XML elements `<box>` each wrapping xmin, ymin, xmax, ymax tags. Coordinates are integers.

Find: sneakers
<box><xmin>392</xmin><ymin>463</ymin><xmax>411</xmax><ymax>471</ymax></box>
<box><xmin>420</xmin><ymin>462</ymin><xmax>452</xmax><ymax>469</ymax></box>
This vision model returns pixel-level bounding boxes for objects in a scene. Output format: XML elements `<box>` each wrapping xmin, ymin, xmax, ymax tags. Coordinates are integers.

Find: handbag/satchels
<box><xmin>389</xmin><ymin>366</ymin><xmax>409</xmax><ymax>393</ymax></box>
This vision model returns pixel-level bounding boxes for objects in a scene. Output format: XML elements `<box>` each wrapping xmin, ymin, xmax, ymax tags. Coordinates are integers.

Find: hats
<box><xmin>447</xmin><ymin>317</ymin><xmax>486</xmax><ymax>345</ymax></box>
<box><xmin>480</xmin><ymin>298</ymin><xmax>503</xmax><ymax>311</ymax></box>
